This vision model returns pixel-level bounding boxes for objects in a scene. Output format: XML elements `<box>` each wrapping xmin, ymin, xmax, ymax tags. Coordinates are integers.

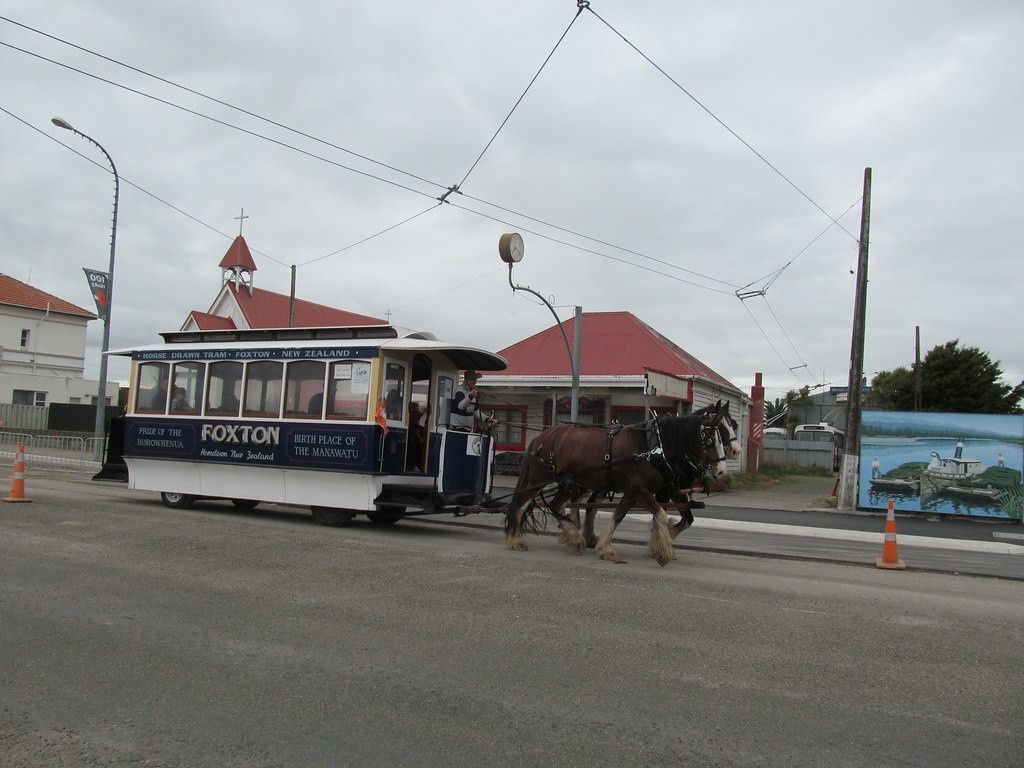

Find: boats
<box><xmin>919</xmin><ymin>437</ymin><xmax>987</xmax><ymax>504</ymax></box>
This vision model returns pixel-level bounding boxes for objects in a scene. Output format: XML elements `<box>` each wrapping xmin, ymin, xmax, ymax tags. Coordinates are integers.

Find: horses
<box><xmin>501</xmin><ymin>400</ymin><xmax>743</xmax><ymax>567</ymax></box>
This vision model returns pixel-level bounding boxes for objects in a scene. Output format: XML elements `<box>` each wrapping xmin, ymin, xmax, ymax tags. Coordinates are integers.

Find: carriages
<box><xmin>92</xmin><ymin>325</ymin><xmax>741</xmax><ymax>566</ymax></box>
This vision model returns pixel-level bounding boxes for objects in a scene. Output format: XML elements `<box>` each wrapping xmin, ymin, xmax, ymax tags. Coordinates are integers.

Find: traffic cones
<box><xmin>10</xmin><ymin>442</ymin><xmax>22</xmax><ymax>478</ymax></box>
<box><xmin>1</xmin><ymin>445</ymin><xmax>33</xmax><ymax>503</ymax></box>
<box><xmin>874</xmin><ymin>500</ymin><xmax>906</xmax><ymax>570</ymax></box>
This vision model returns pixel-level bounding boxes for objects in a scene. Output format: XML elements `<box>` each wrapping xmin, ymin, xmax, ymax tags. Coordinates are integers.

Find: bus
<box><xmin>794</xmin><ymin>422</ymin><xmax>845</xmax><ymax>472</ymax></box>
<box><xmin>763</xmin><ymin>428</ymin><xmax>789</xmax><ymax>440</ymax></box>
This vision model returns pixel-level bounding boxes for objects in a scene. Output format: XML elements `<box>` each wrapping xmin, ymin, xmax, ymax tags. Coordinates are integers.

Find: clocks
<box><xmin>509</xmin><ymin>233</ymin><xmax>524</xmax><ymax>261</ymax></box>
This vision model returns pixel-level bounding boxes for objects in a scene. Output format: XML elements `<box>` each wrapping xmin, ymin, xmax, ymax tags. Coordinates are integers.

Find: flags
<box><xmin>375</xmin><ymin>400</ymin><xmax>389</xmax><ymax>435</ymax></box>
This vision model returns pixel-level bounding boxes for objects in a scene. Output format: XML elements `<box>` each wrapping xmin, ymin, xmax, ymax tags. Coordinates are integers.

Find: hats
<box><xmin>464</xmin><ymin>370</ymin><xmax>482</xmax><ymax>380</ymax></box>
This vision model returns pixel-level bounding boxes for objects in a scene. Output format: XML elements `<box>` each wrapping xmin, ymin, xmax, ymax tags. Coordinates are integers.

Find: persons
<box><xmin>385</xmin><ymin>390</ymin><xmax>434</xmax><ymax>471</ymax></box>
<box><xmin>153</xmin><ymin>373</ymin><xmax>193</xmax><ymax>410</ymax></box>
<box><xmin>872</xmin><ymin>456</ymin><xmax>882</xmax><ymax>479</ymax></box>
<box><xmin>997</xmin><ymin>452</ymin><xmax>1006</xmax><ymax>469</ymax></box>
<box><xmin>218</xmin><ymin>392</ymin><xmax>238</xmax><ymax>412</ymax></box>
<box><xmin>449</xmin><ymin>371</ymin><xmax>498</xmax><ymax>433</ymax></box>
<box><xmin>172</xmin><ymin>387</ymin><xmax>187</xmax><ymax>410</ymax></box>
<box><xmin>308</xmin><ymin>380</ymin><xmax>350</xmax><ymax>416</ymax></box>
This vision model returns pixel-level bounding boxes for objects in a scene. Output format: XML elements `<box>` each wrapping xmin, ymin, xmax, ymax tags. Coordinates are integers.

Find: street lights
<box><xmin>51</xmin><ymin>117</ymin><xmax>119</xmax><ymax>463</ymax></box>
